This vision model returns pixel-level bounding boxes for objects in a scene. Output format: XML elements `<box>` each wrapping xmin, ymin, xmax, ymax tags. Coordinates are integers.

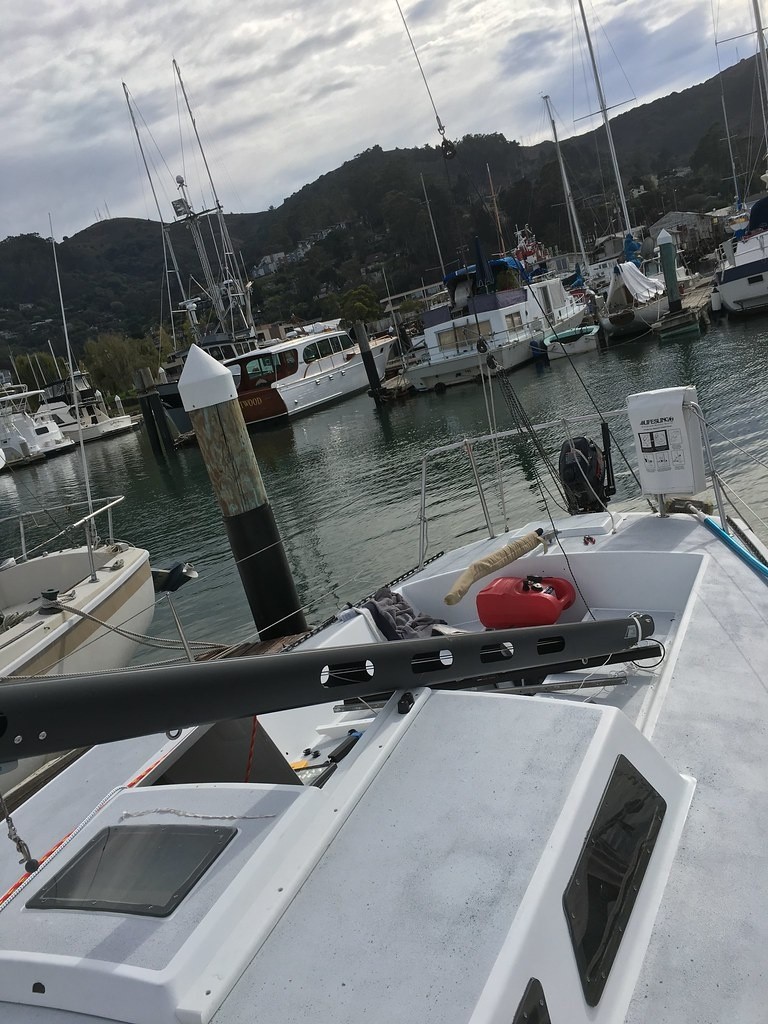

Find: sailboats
<box><xmin>122</xmin><ymin>60</ymin><xmax>258</xmax><ymax>410</ymax></box>
<box><xmin>0</xmin><ymin>413</ymin><xmax>768</xmax><ymax>1024</ymax></box>
<box><xmin>0</xmin><ymin>339</ymin><xmax>139</xmax><ymax>468</ymax></box>
<box><xmin>0</xmin><ymin>212</ymin><xmax>156</xmax><ymax>811</ymax></box>
<box><xmin>396</xmin><ymin>0</ymin><xmax>768</xmax><ymax>391</ymax></box>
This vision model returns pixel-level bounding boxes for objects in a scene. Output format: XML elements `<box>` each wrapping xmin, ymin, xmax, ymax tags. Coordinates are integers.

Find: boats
<box><xmin>223</xmin><ymin>331</ymin><xmax>396</xmax><ymax>425</ymax></box>
